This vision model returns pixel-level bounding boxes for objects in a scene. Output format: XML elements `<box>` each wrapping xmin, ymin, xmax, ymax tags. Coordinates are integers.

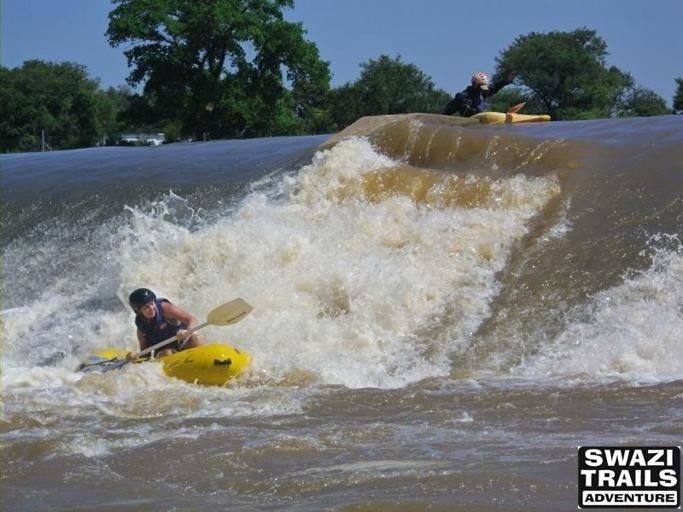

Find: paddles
<box><xmin>104</xmin><ymin>297</ymin><xmax>253</xmax><ymax>373</ymax></box>
<box><xmin>509</xmin><ymin>102</ymin><xmax>526</xmax><ymax>112</ymax></box>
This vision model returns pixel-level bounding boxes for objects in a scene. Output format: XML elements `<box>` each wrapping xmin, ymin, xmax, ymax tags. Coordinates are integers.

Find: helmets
<box><xmin>471</xmin><ymin>71</ymin><xmax>489</xmax><ymax>90</ymax></box>
<box><xmin>128</xmin><ymin>287</ymin><xmax>157</xmax><ymax>314</ymax></box>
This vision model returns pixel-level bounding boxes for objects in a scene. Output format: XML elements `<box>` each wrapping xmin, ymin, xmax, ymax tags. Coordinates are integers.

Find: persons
<box><xmin>442</xmin><ymin>72</ymin><xmax>489</xmax><ymax>118</ymax></box>
<box><xmin>124</xmin><ymin>289</ymin><xmax>200</xmax><ymax>360</ymax></box>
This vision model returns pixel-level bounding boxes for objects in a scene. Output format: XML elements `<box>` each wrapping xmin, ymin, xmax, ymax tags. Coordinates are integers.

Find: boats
<box><xmin>72</xmin><ymin>343</ymin><xmax>255</xmax><ymax>413</ymax></box>
<box><xmin>467</xmin><ymin>110</ymin><xmax>551</xmax><ymax>125</ymax></box>
